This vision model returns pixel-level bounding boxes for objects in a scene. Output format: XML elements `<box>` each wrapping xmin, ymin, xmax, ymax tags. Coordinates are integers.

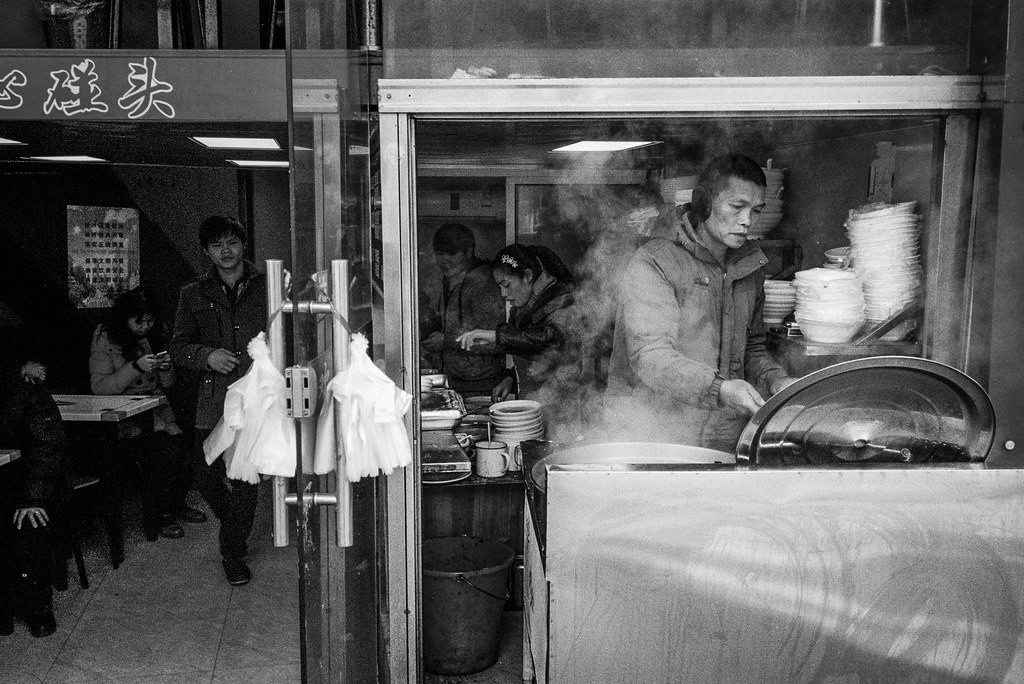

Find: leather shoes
<box><xmin>28</xmin><ymin>600</ymin><xmax>56</xmax><ymax>636</ymax></box>
<box><xmin>159</xmin><ymin>512</ymin><xmax>184</xmax><ymax>538</ymax></box>
<box><xmin>181</xmin><ymin>506</ymin><xmax>207</xmax><ymax>523</ymax></box>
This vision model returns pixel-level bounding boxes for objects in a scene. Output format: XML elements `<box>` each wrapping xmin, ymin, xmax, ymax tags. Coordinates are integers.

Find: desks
<box><xmin>48</xmin><ymin>392</ymin><xmax>170</xmax><ymax>570</ymax></box>
<box><xmin>0</xmin><ymin>449</ymin><xmax>31</xmax><ymax>637</ymax></box>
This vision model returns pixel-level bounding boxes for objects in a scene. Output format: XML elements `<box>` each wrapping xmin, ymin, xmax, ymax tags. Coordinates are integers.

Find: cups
<box><xmin>475</xmin><ymin>441</ymin><xmax>510</xmax><ymax>478</ymax></box>
<box><xmin>513</xmin><ymin>439</ymin><xmax>550</xmax><ymax>475</ymax></box>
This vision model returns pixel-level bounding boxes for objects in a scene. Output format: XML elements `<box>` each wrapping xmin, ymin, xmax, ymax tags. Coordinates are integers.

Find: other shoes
<box><xmin>240</xmin><ymin>540</ymin><xmax>248</xmax><ymax>559</ymax></box>
<box><xmin>221</xmin><ymin>555</ymin><xmax>249</xmax><ymax>584</ymax></box>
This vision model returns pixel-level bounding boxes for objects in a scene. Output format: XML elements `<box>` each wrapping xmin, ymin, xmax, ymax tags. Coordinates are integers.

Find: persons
<box><xmin>422</xmin><ymin>225</ymin><xmax>504</xmax><ymax>395</ymax></box>
<box><xmin>456</xmin><ymin>243</ymin><xmax>577</xmax><ymax>402</ymax></box>
<box><xmin>89</xmin><ymin>285</ymin><xmax>205</xmax><ymax>536</ymax></box>
<box><xmin>0</xmin><ymin>359</ymin><xmax>67</xmax><ymax>637</ymax></box>
<box><xmin>604</xmin><ymin>154</ymin><xmax>802</xmax><ymax>451</ymax></box>
<box><xmin>169</xmin><ymin>217</ymin><xmax>294</xmax><ymax>585</ymax></box>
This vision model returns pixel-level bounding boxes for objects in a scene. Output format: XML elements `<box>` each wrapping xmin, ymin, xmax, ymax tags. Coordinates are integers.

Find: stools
<box><xmin>14</xmin><ymin>420</ymin><xmax>193</xmax><ymax>591</ymax></box>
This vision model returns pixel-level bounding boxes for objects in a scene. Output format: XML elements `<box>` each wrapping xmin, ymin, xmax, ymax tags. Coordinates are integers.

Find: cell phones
<box><xmin>155</xmin><ymin>351</ymin><xmax>168</xmax><ymax>359</ymax></box>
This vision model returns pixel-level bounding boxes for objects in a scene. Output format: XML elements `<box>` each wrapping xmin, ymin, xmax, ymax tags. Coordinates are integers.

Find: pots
<box><xmin>525</xmin><ymin>442</ymin><xmax>737</xmax><ymax>494</ymax></box>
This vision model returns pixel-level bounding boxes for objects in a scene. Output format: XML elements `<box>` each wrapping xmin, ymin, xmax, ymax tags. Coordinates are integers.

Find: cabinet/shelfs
<box><xmin>605</xmin><ymin>232</ymin><xmax>794</xmax><ymax>332</ymax></box>
<box><xmin>761</xmin><ymin>328</ymin><xmax>926</xmax><ymax>386</ymax></box>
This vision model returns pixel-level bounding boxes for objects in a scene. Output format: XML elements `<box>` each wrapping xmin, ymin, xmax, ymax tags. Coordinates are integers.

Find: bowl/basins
<box><xmin>745</xmin><ymin>166</ymin><xmax>924</xmax><ymax>343</ymax></box>
<box><xmin>489</xmin><ymin>399</ymin><xmax>544</xmax><ymax>472</ymax></box>
<box><xmin>660</xmin><ymin>174</ymin><xmax>700</xmax><ymax>205</ymax></box>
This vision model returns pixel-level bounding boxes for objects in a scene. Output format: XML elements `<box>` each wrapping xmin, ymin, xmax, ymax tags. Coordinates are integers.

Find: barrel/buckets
<box><xmin>421</xmin><ymin>534</ymin><xmax>516</xmax><ymax>675</ymax></box>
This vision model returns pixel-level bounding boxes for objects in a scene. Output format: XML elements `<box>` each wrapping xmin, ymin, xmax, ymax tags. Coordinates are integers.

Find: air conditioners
<box><xmin>417</xmin><ymin>187</ymin><xmax>505</xmax><ymax>225</ymax></box>
